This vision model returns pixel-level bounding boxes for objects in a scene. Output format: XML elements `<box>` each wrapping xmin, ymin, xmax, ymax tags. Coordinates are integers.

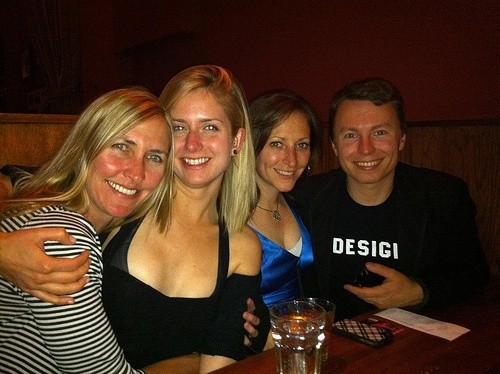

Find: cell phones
<box><xmin>332</xmin><ymin>319</ymin><xmax>393</xmax><ymax>348</ymax></box>
<box><xmin>353</xmin><ymin>268</ymin><xmax>384</xmax><ymax>287</ymax></box>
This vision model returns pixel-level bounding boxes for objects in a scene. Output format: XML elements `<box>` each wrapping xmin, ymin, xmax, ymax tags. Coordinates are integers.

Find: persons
<box><xmin>283</xmin><ymin>75</ymin><xmax>492</xmax><ymax>325</ymax></box>
<box><xmin>0</xmin><ymin>64</ymin><xmax>324</xmax><ymax>374</ymax></box>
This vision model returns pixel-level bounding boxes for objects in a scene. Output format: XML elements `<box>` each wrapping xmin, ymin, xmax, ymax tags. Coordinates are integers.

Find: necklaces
<box><xmin>254</xmin><ymin>194</ymin><xmax>283</xmax><ymax>224</ymax></box>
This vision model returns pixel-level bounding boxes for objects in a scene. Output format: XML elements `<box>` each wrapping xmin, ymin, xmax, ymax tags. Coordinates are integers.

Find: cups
<box><xmin>269</xmin><ymin>300</ymin><xmax>326</xmax><ymax>374</ymax></box>
<box><xmin>288</xmin><ymin>298</ymin><xmax>336</xmax><ymax>361</ymax></box>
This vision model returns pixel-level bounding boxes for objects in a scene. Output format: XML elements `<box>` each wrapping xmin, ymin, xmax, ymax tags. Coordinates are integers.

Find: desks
<box><xmin>207</xmin><ymin>274</ymin><xmax>500</xmax><ymax>374</ymax></box>
